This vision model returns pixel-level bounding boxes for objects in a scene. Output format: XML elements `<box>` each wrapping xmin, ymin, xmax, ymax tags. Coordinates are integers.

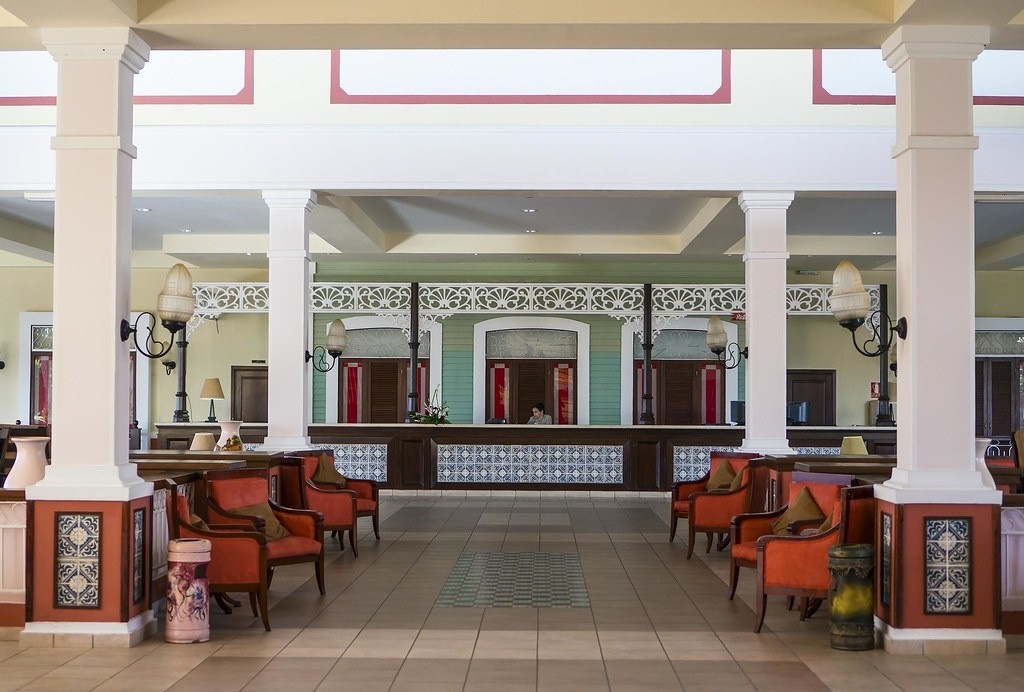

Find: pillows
<box><xmin>817</xmin><ymin>510</ymin><xmax>834</xmax><ymax>537</ymax></box>
<box><xmin>769</xmin><ymin>486</ymin><xmax>826</xmax><ymax>537</ymax></box>
<box><xmin>705</xmin><ymin>459</ymin><xmax>735</xmax><ymax>490</ymax></box>
<box><xmin>314</xmin><ymin>452</ymin><xmax>346</xmax><ymax>488</ymax></box>
<box><xmin>190</xmin><ymin>514</ymin><xmax>210</xmax><ymax>532</ymax></box>
<box><xmin>229</xmin><ymin>501</ymin><xmax>292</xmax><ymax>542</ymax></box>
<box><xmin>731</xmin><ymin>463</ymin><xmax>748</xmax><ymax>493</ymax></box>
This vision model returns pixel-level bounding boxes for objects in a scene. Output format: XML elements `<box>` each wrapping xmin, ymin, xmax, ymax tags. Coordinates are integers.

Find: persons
<box><xmin>526</xmin><ymin>402</ymin><xmax>553</xmax><ymax>425</ymax></box>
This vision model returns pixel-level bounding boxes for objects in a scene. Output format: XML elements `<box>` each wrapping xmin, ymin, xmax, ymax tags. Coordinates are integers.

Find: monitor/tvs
<box><xmin>787</xmin><ymin>401</ymin><xmax>808</xmax><ymax>423</ymax></box>
<box><xmin>731</xmin><ymin>401</ymin><xmax>745</xmax><ymax>426</ymax></box>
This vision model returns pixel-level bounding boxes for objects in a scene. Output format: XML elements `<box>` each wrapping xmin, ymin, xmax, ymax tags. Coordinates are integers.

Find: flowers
<box><xmin>407</xmin><ymin>385</ymin><xmax>453</xmax><ymax>425</ymax></box>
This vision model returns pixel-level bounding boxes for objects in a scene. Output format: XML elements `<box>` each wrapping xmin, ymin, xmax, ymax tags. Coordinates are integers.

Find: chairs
<box><xmin>668</xmin><ymin>450</ymin><xmax>874</xmax><ymax>634</ymax></box>
<box><xmin>164</xmin><ymin>450</ymin><xmax>383</xmax><ymax>632</ymax></box>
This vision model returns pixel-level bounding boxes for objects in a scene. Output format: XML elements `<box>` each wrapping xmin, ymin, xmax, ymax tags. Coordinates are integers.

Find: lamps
<box><xmin>305</xmin><ymin>319</ymin><xmax>346</xmax><ymax>372</ymax></box>
<box><xmin>706</xmin><ymin>316</ymin><xmax>748</xmax><ymax>369</ymax></box>
<box><xmin>199</xmin><ymin>378</ymin><xmax>225</xmax><ymax>423</ymax></box>
<box><xmin>889</xmin><ymin>343</ymin><xmax>897</xmax><ymax>379</ymax></box>
<box><xmin>827</xmin><ymin>260</ymin><xmax>908</xmax><ymax>357</ymax></box>
<box><xmin>120</xmin><ymin>263</ymin><xmax>195</xmax><ymax>376</ymax></box>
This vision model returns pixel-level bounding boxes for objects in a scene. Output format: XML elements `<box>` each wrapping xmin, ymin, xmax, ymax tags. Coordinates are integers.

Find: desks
<box><xmin>129</xmin><ymin>449</ymin><xmax>284</xmax><ymax>504</ymax></box>
